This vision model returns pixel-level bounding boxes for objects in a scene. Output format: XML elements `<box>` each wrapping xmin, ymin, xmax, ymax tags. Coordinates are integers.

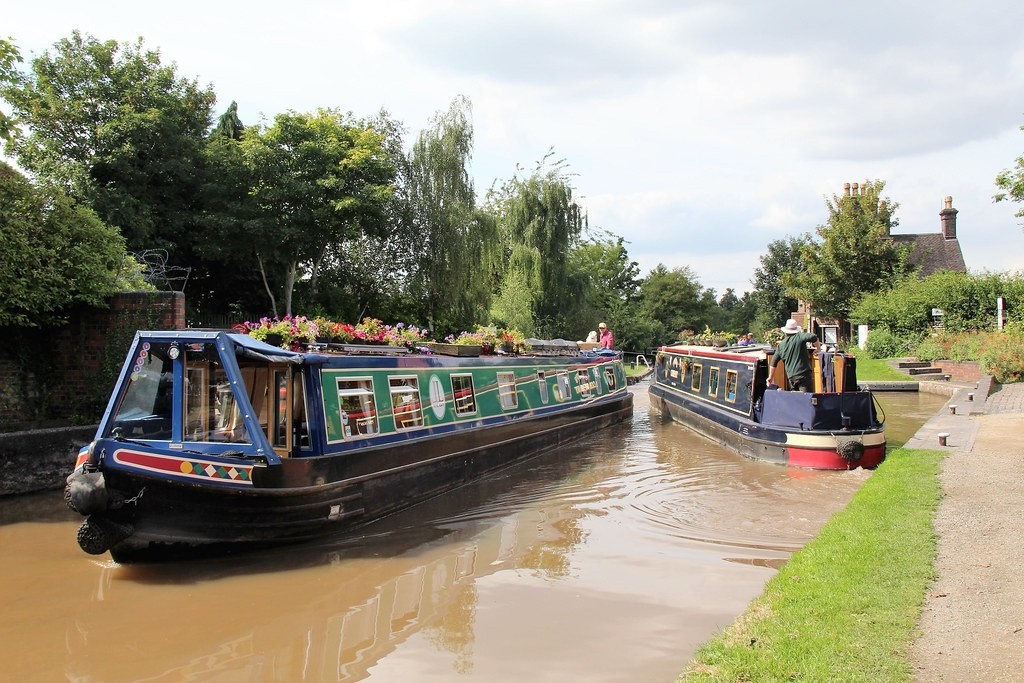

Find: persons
<box><xmin>598</xmin><ymin>323</ymin><xmax>613</xmax><ymax>350</ymax></box>
<box><xmin>738</xmin><ymin>333</ymin><xmax>754</xmax><ymax>346</ymax></box>
<box><xmin>766</xmin><ymin>319</ymin><xmax>821</xmax><ymax>393</ymax></box>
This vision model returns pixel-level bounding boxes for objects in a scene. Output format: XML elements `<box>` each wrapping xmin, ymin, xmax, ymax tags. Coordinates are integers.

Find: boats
<box><xmin>650</xmin><ymin>326</ymin><xmax>886</xmax><ymax>470</ymax></box>
<box><xmin>66</xmin><ymin>330</ymin><xmax>632</xmax><ymax>566</ymax></box>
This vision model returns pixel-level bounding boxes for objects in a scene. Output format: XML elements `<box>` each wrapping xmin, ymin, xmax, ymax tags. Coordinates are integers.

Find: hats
<box><xmin>598</xmin><ymin>322</ymin><xmax>607</xmax><ymax>329</ymax></box>
<box><xmin>741</xmin><ymin>335</ymin><xmax>747</xmax><ymax>339</ymax></box>
<box><xmin>747</xmin><ymin>332</ymin><xmax>753</xmax><ymax>335</ymax></box>
<box><xmin>781</xmin><ymin>319</ymin><xmax>801</xmax><ymax>334</ymax></box>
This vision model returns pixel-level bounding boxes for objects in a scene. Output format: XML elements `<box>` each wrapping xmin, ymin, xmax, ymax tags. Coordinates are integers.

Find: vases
<box><xmin>774</xmin><ymin>341</ymin><xmax>783</xmax><ymax>349</ymax></box>
<box><xmin>290</xmin><ymin>336</ymin><xmax>310</xmax><ymax>351</ymax></box>
<box><xmin>682</xmin><ymin>341</ymin><xmax>687</xmax><ymax>345</ymax></box>
<box><xmin>265</xmin><ymin>333</ymin><xmax>283</xmax><ymax>348</ymax></box>
<box><xmin>431</xmin><ymin>344</ymin><xmax>482</xmax><ymax>357</ymax></box>
<box><xmin>498</xmin><ymin>341</ymin><xmax>514</xmax><ymax>356</ymax></box>
<box><xmin>483</xmin><ymin>344</ymin><xmax>494</xmax><ymax>356</ymax></box>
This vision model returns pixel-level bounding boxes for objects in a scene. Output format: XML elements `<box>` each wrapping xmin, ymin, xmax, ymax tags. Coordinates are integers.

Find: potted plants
<box><xmin>726</xmin><ymin>333</ymin><xmax>738</xmax><ymax>347</ymax></box>
<box><xmin>656</xmin><ymin>352</ymin><xmax>665</xmax><ymax>363</ymax></box>
<box><xmin>692</xmin><ymin>334</ymin><xmax>703</xmax><ymax>346</ymax></box>
<box><xmin>687</xmin><ymin>336</ymin><xmax>695</xmax><ymax>345</ymax></box>
<box><xmin>703</xmin><ymin>324</ymin><xmax>713</xmax><ymax>346</ymax></box>
<box><xmin>682</xmin><ymin>357</ymin><xmax>690</xmax><ymax>371</ymax></box>
<box><xmin>698</xmin><ymin>334</ymin><xmax>706</xmax><ymax>346</ymax></box>
<box><xmin>712</xmin><ymin>331</ymin><xmax>727</xmax><ymax>347</ymax></box>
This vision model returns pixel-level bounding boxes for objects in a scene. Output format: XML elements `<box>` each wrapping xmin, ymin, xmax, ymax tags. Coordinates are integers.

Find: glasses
<box><xmin>600</xmin><ymin>327</ymin><xmax>605</xmax><ymax>329</ymax></box>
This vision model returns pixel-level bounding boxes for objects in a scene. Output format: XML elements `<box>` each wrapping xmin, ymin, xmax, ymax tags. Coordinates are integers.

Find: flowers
<box><xmin>239</xmin><ymin>316</ymin><xmax>436</xmax><ymax>354</ymax></box>
<box><xmin>678</xmin><ymin>329</ymin><xmax>694</xmax><ymax>343</ymax></box>
<box><xmin>762</xmin><ymin>327</ymin><xmax>785</xmax><ymax>349</ymax></box>
<box><xmin>445</xmin><ymin>325</ymin><xmax>531</xmax><ymax>353</ymax></box>
